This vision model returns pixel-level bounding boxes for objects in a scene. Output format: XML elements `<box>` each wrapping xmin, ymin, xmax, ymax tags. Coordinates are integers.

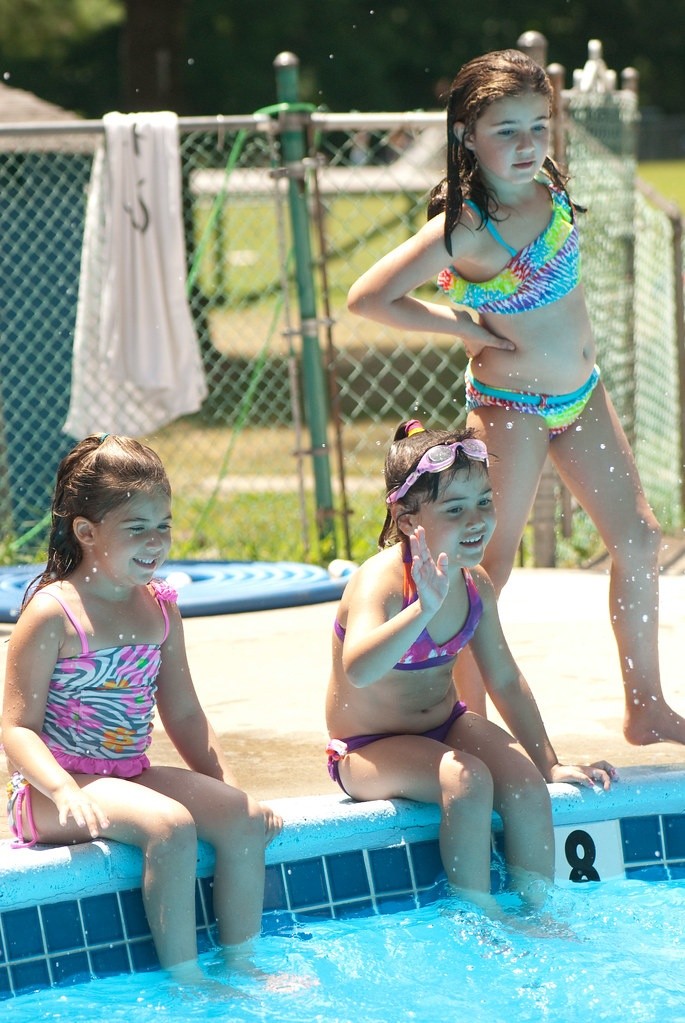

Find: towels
<box><xmin>59</xmin><ymin>109</ymin><xmax>209</xmax><ymax>447</ymax></box>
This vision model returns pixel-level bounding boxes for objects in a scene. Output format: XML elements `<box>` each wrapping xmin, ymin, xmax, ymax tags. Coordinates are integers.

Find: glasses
<box><xmin>385</xmin><ymin>438</ymin><xmax>488</xmax><ymax>504</ymax></box>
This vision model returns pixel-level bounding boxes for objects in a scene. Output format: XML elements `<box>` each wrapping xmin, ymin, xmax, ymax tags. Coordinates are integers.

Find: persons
<box><xmin>3</xmin><ymin>433</ymin><xmax>282</xmax><ymax>1001</ymax></box>
<box><xmin>323</xmin><ymin>418</ymin><xmax>573</xmax><ymax>941</ymax></box>
<box><xmin>345</xmin><ymin>51</ymin><xmax>685</xmax><ymax>749</ymax></box>
